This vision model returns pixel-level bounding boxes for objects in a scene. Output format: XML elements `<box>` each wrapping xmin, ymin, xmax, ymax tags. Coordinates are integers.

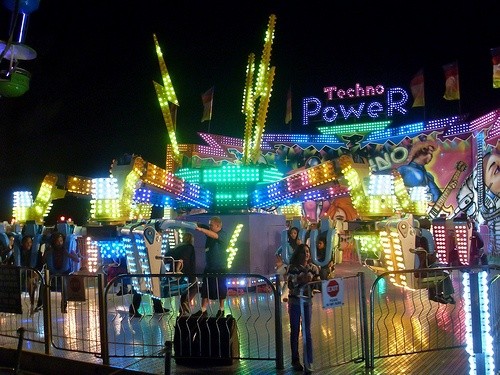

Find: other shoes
<box><xmin>35</xmin><ymin>305</ymin><xmax>43</xmax><ymax>311</ymax></box>
<box><xmin>304</xmin><ymin>363</ymin><xmax>311</xmax><ymax>375</ymax></box>
<box><xmin>216</xmin><ymin>309</ymin><xmax>223</xmax><ymax>318</ymax></box>
<box><xmin>129</xmin><ymin>312</ymin><xmax>142</xmax><ymax>318</ymax></box>
<box><xmin>192</xmin><ymin>310</ymin><xmax>208</xmax><ymax>317</ymax></box>
<box><xmin>156</xmin><ymin>308</ymin><xmax>169</xmax><ymax>313</ymax></box>
<box><xmin>441</xmin><ymin>295</ymin><xmax>458</xmax><ymax>304</ymax></box>
<box><xmin>291</xmin><ymin>359</ymin><xmax>303</xmax><ymax>370</ymax></box>
<box><xmin>429</xmin><ymin>297</ymin><xmax>440</xmax><ymax>302</ymax></box>
<box><xmin>62</xmin><ymin>308</ymin><xmax>67</xmax><ymax>313</ymax></box>
<box><xmin>116</xmin><ymin>286</ymin><xmax>128</xmax><ymax>296</ymax></box>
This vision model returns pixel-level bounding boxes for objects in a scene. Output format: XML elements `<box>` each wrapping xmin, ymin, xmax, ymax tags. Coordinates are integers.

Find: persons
<box><xmin>21</xmin><ymin>236</ymin><xmax>42</xmax><ymax>305</ymax></box>
<box><xmin>305</xmin><ymin>229</ymin><xmax>336</xmax><ymax>294</ymax></box>
<box><xmin>129</xmin><ymin>222</ymin><xmax>170</xmax><ymax>318</ymax></box>
<box><xmin>0</xmin><ymin>221</ymin><xmax>15</xmax><ymax>265</ymax></box>
<box><xmin>164</xmin><ymin>233</ymin><xmax>199</xmax><ymax>315</ymax></box>
<box><xmin>116</xmin><ymin>277</ymin><xmax>136</xmax><ymax>296</ymax></box>
<box><xmin>469</xmin><ymin>226</ymin><xmax>488</xmax><ymax>265</ymax></box>
<box><xmin>287</xmin><ymin>243</ymin><xmax>321</xmax><ymax>373</ymax></box>
<box><xmin>274</xmin><ymin>227</ymin><xmax>303</xmax><ymax>302</ymax></box>
<box><xmin>192</xmin><ymin>216</ymin><xmax>227</xmax><ymax>318</ymax></box>
<box><xmin>414</xmin><ymin>219</ymin><xmax>457</xmax><ymax>304</ymax></box>
<box><xmin>33</xmin><ymin>233</ymin><xmax>80</xmax><ymax>313</ymax></box>
